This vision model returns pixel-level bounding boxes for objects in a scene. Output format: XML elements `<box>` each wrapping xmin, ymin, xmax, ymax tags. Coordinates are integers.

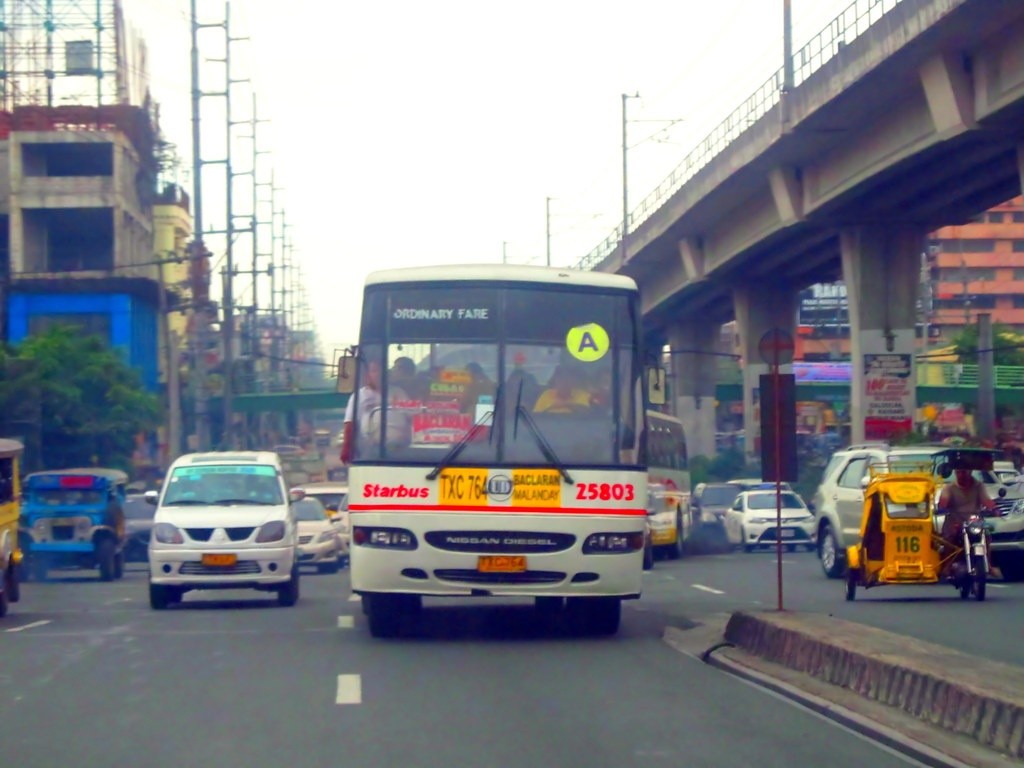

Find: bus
<box><xmin>329</xmin><ymin>265</ymin><xmax>653</xmax><ymax>635</ymax></box>
<box><xmin>641</xmin><ymin>407</ymin><xmax>694</xmax><ymax>545</ymax></box>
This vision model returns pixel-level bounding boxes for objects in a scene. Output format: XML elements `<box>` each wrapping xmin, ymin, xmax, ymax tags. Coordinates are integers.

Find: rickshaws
<box><xmin>842</xmin><ymin>452</ymin><xmax>993</xmax><ymax>597</ymax></box>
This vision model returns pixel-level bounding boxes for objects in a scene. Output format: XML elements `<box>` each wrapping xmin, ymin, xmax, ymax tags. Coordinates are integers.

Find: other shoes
<box><xmin>943</xmin><ymin>566</ymin><xmax>954</xmax><ymax>577</ymax></box>
<box><xmin>989</xmin><ymin>566</ymin><xmax>1002</xmax><ymax>580</ymax></box>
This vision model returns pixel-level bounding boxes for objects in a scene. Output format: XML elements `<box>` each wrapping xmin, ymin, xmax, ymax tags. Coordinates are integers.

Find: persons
<box><xmin>389</xmin><ymin>356</ymin><xmax>543</xmax><ymax>415</ymax></box>
<box><xmin>533</xmin><ymin>365</ymin><xmax>601</xmax><ymax>413</ymax></box>
<box><xmin>937</xmin><ymin>467</ymin><xmax>1002</xmax><ymax>575</ymax></box>
<box><xmin>339</xmin><ymin>360</ymin><xmax>410</xmax><ymax>466</ymax></box>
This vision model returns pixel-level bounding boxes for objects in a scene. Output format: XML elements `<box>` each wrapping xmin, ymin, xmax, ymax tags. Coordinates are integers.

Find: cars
<box><xmin>726</xmin><ymin>491</ymin><xmax>818</xmax><ymax>551</ymax></box>
<box><xmin>120</xmin><ymin>489</ymin><xmax>157</xmax><ymax>557</ymax></box>
<box><xmin>24</xmin><ymin>465</ymin><xmax>127</xmax><ymax>578</ymax></box>
<box><xmin>692</xmin><ymin>482</ymin><xmax>744</xmax><ymax>551</ymax></box>
<box><xmin>813</xmin><ymin>446</ymin><xmax>1022</xmax><ymax>575</ymax></box>
<box><xmin>649</xmin><ymin>490</ymin><xmax>690</xmax><ymax>545</ymax></box>
<box><xmin>284</xmin><ymin>488</ymin><xmax>352</xmax><ymax>568</ymax></box>
<box><xmin>0</xmin><ymin>440</ymin><xmax>25</xmax><ymax>616</ymax></box>
<box><xmin>149</xmin><ymin>449</ymin><xmax>301</xmax><ymax>603</ymax></box>
